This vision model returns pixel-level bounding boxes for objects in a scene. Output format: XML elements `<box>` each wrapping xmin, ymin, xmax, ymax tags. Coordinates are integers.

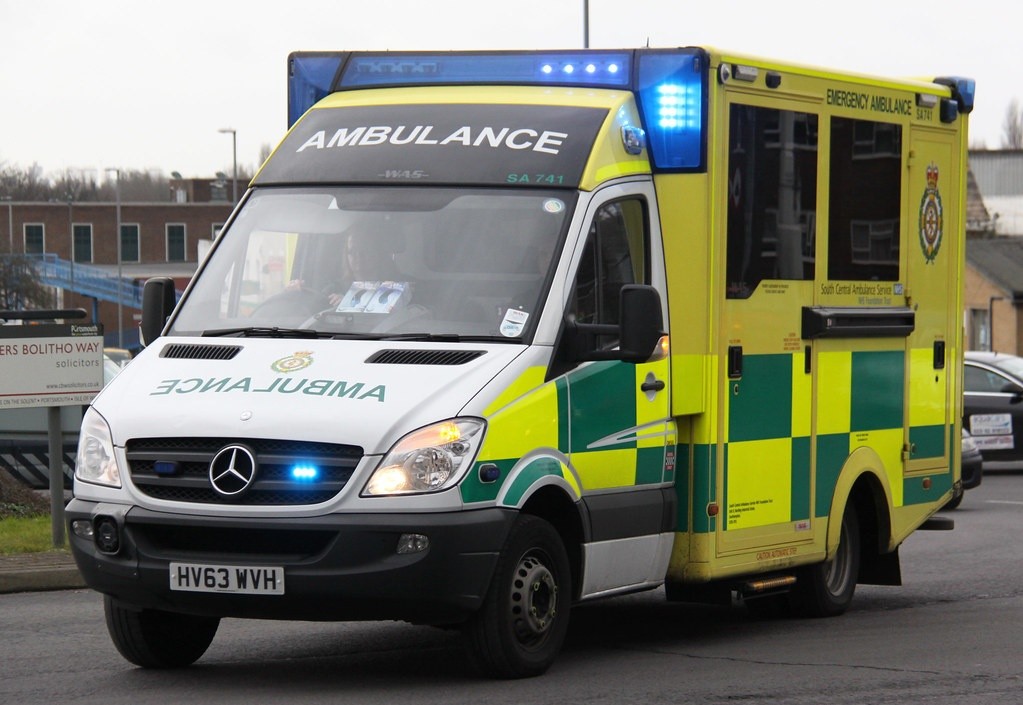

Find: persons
<box><xmin>287</xmin><ymin>226</ymin><xmax>414</xmax><ymax>321</ymax></box>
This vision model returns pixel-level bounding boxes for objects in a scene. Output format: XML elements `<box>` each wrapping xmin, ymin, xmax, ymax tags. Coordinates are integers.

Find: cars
<box><xmin>939</xmin><ymin>428</ymin><xmax>982</xmax><ymax>511</ymax></box>
<box><xmin>963</xmin><ymin>351</ymin><xmax>1023</xmax><ymax>462</ymax></box>
<box><xmin>102</xmin><ymin>347</ymin><xmax>131</xmax><ymax>366</ymax></box>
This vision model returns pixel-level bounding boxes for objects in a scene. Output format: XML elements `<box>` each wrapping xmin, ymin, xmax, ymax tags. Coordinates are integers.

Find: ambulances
<box><xmin>64</xmin><ymin>49</ymin><xmax>977</xmax><ymax>678</ymax></box>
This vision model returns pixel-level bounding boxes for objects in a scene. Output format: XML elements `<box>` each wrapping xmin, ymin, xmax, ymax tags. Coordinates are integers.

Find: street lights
<box><xmin>218</xmin><ymin>128</ymin><xmax>238</xmax><ymax>211</ymax></box>
<box><xmin>104</xmin><ymin>166</ymin><xmax>124</xmax><ymax>350</ymax></box>
